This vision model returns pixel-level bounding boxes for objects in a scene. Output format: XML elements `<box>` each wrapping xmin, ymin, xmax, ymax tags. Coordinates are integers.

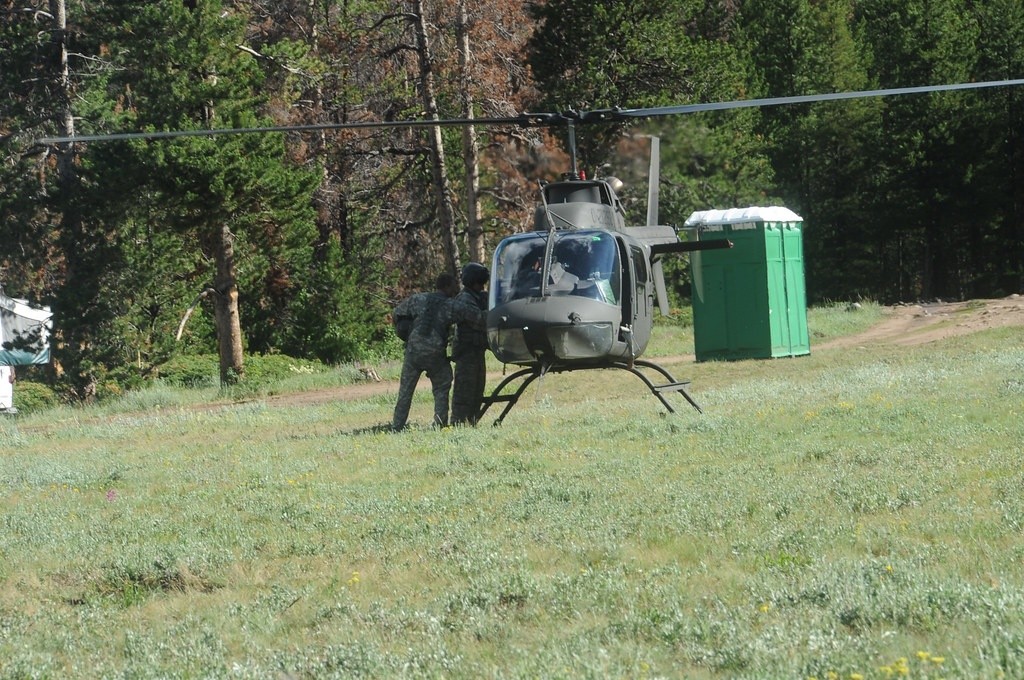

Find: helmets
<box><xmin>461</xmin><ymin>262</ymin><xmax>489</xmax><ymax>282</ymax></box>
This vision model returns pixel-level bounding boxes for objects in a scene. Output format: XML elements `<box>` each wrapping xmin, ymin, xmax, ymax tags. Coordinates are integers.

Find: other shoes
<box><xmin>392</xmin><ymin>426</ymin><xmax>405</xmax><ymax>434</ymax></box>
<box><xmin>450</xmin><ymin>418</ymin><xmax>475</xmax><ymax>428</ymax></box>
<box><xmin>433</xmin><ymin>422</ymin><xmax>447</xmax><ymax>432</ymax></box>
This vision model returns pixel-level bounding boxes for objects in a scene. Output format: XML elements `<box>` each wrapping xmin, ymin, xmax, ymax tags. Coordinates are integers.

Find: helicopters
<box><xmin>40</xmin><ymin>84</ymin><xmax>1024</xmax><ymax>432</ymax></box>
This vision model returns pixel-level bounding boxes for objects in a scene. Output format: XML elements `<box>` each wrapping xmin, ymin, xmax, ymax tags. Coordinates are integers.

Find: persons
<box><xmin>391</xmin><ymin>271</ymin><xmax>464</xmax><ymax>430</ymax></box>
<box><xmin>532</xmin><ymin>257</ymin><xmax>542</xmax><ymax>273</ymax></box>
<box><xmin>451</xmin><ymin>262</ymin><xmax>490</xmax><ymax>427</ymax></box>
